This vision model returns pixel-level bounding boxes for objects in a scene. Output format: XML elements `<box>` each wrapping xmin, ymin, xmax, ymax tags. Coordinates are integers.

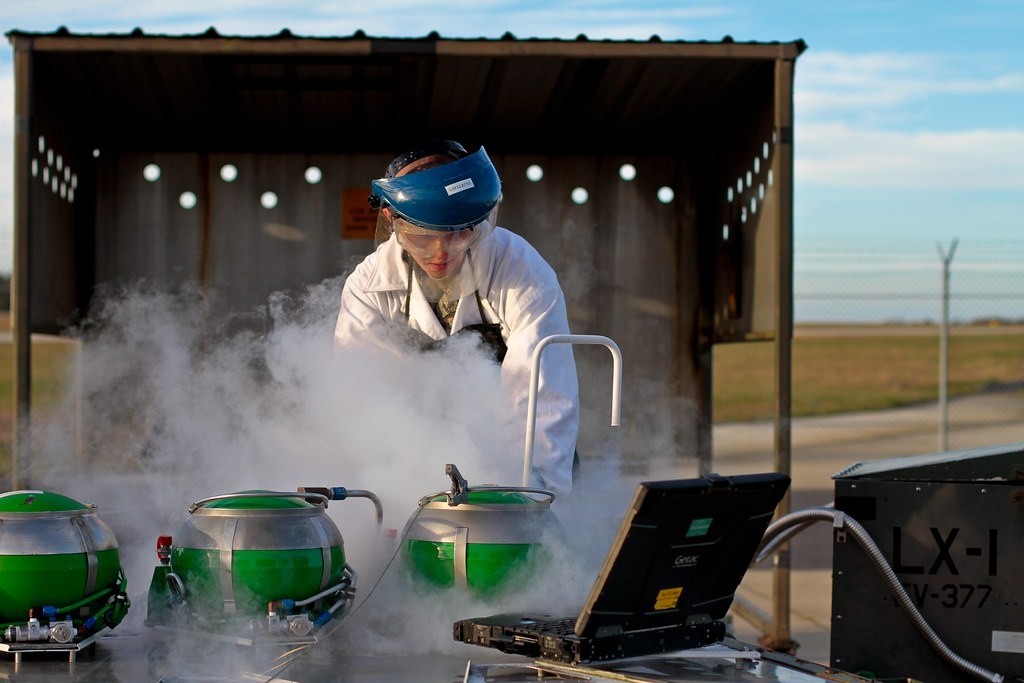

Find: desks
<box><xmin>0</xmin><ymin>624</ymin><xmax>884</xmax><ymax>683</ymax></box>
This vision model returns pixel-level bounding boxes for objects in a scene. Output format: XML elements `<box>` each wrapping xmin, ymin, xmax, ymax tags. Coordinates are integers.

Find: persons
<box><xmin>333</xmin><ymin>137</ymin><xmax>583</xmax><ymax>503</ymax></box>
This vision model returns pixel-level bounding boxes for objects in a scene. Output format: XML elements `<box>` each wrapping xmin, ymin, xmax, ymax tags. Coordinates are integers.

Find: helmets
<box><xmin>371</xmin><ymin>138</ymin><xmax>502</xmax><ymax>231</ymax></box>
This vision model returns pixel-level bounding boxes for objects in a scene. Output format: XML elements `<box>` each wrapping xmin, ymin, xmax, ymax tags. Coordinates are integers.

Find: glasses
<box><xmin>387</xmin><ymin>218</ymin><xmax>481</xmax><ymax>258</ymax></box>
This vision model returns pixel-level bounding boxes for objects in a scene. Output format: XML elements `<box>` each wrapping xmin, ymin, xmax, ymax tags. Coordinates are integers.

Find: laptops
<box><xmin>452</xmin><ymin>472</ymin><xmax>790</xmax><ymax>663</ymax></box>
